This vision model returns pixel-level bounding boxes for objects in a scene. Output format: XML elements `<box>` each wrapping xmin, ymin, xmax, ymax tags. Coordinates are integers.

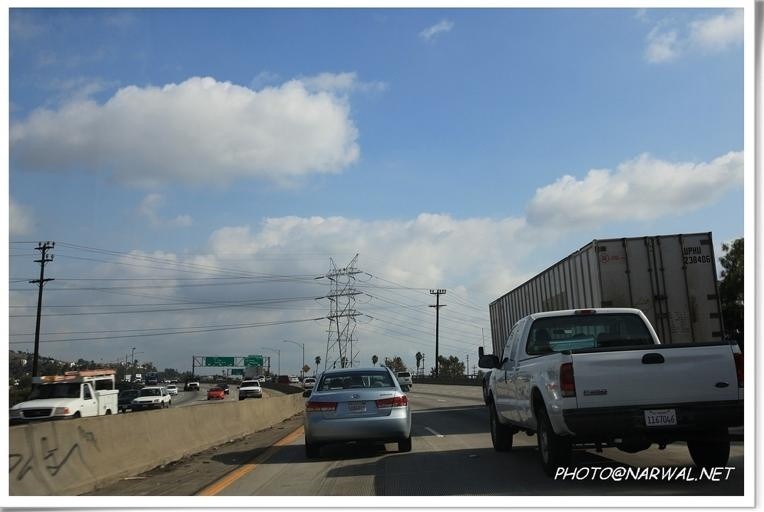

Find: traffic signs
<box><xmin>243</xmin><ymin>354</ymin><xmax>263</xmax><ymax>367</ymax></box>
<box><xmin>206</xmin><ymin>357</ymin><xmax>234</xmax><ymax>367</ymax></box>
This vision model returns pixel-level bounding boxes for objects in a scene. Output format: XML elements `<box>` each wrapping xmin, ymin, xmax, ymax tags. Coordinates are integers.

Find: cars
<box><xmin>184</xmin><ymin>377</ymin><xmax>199</xmax><ymax>390</ymax></box>
<box><xmin>200</xmin><ymin>375</ymin><xmax>299</xmax><ymax>400</ymax></box>
<box><xmin>119</xmin><ymin>372</ymin><xmax>178</xmax><ymax>412</ymax></box>
<box><xmin>302</xmin><ymin>366</ymin><xmax>412</xmax><ymax>458</ymax></box>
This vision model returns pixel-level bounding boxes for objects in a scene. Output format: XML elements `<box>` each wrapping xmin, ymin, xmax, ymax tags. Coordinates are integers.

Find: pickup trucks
<box><xmin>479</xmin><ymin>308</ymin><xmax>744</xmax><ymax>479</ymax></box>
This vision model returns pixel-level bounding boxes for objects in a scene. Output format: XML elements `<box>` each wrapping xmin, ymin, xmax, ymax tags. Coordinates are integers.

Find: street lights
<box><xmin>283</xmin><ymin>340</ymin><xmax>304</xmax><ymax>378</ymax></box>
<box><xmin>262</xmin><ymin>347</ymin><xmax>280</xmax><ymax>375</ymax></box>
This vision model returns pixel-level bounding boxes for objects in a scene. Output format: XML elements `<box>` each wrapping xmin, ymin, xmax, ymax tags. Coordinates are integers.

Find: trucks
<box><xmin>482</xmin><ymin>231</ymin><xmax>728</xmax><ymax>407</ymax></box>
<box><xmin>10</xmin><ymin>374</ymin><xmax>119</xmax><ymax>423</ymax></box>
